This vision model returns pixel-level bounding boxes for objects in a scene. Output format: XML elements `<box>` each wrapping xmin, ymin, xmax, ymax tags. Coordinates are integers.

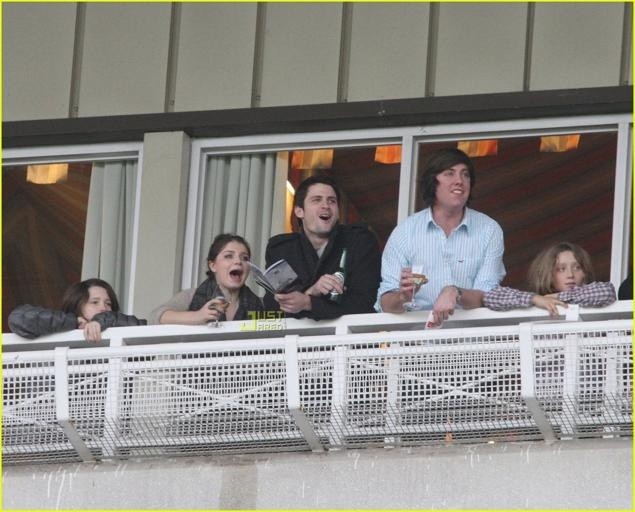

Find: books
<box><xmin>248</xmin><ymin>259</ymin><xmax>298</xmax><ymax>295</ymax></box>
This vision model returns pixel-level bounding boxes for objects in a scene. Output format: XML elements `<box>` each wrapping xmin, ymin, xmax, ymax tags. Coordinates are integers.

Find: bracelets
<box><xmin>449</xmin><ymin>285</ymin><xmax>462</xmax><ymax>302</ymax></box>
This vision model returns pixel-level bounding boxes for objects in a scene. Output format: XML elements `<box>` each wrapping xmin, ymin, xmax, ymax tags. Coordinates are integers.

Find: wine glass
<box><xmin>401</xmin><ymin>252</ymin><xmax>426</xmax><ymax>312</ymax></box>
<box><xmin>204</xmin><ymin>282</ymin><xmax>232</xmax><ymax>330</ymax></box>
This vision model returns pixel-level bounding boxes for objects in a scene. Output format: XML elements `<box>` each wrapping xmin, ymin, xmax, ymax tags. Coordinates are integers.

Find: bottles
<box><xmin>325</xmin><ymin>245</ymin><xmax>351</xmax><ymax>303</ymax></box>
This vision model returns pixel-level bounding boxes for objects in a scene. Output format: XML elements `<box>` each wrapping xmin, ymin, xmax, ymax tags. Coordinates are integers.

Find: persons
<box><xmin>373</xmin><ymin>149</ymin><xmax>506</xmax><ymax>326</ymax></box>
<box><xmin>263</xmin><ymin>177</ymin><xmax>381</xmax><ymax>320</ymax></box>
<box><xmin>146</xmin><ymin>234</ymin><xmax>263</xmax><ymax>326</ymax></box>
<box><xmin>482</xmin><ymin>241</ymin><xmax>615</xmax><ymax>317</ymax></box>
<box><xmin>7</xmin><ymin>279</ymin><xmax>147</xmax><ymax>344</ymax></box>
